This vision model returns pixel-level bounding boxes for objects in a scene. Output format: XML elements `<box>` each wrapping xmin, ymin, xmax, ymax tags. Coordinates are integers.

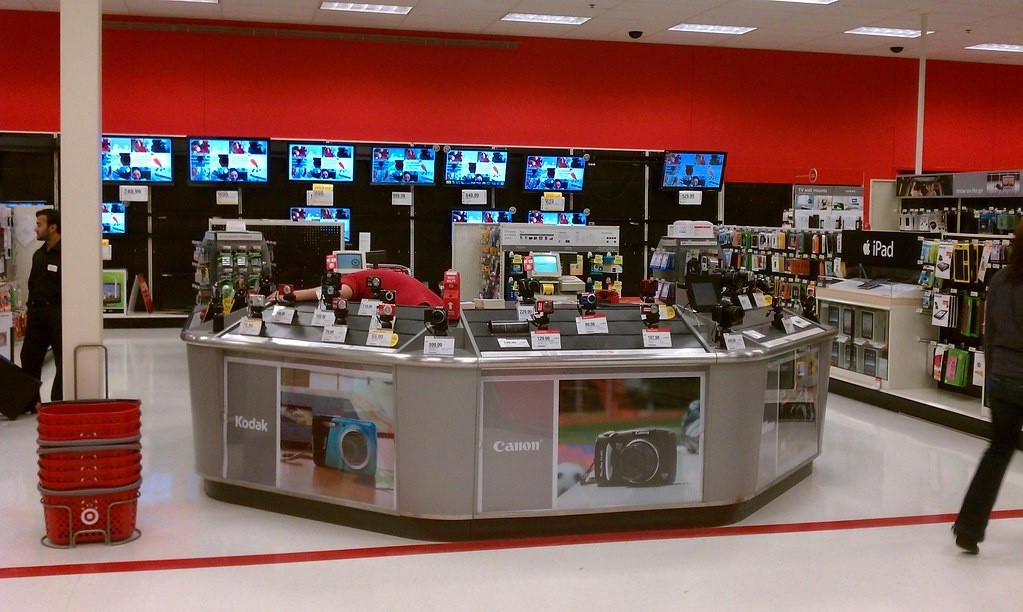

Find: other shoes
<box><xmin>33</xmin><ymin>402</ymin><xmax>40</xmax><ymax>413</ymax></box>
<box><xmin>957</xmin><ymin>533</ymin><xmax>979</xmax><ymax>554</ymax></box>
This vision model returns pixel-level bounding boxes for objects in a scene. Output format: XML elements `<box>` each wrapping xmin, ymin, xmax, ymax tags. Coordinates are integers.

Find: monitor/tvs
<box><xmin>530</xmin><ymin>253</ymin><xmax>562</xmax><ymax>277</ymax></box>
<box><xmin>452</xmin><ymin>209</ymin><xmax>512</xmax><ymax>223</ymax></box>
<box><xmin>660</xmin><ymin>150</ymin><xmax>727</xmax><ymax>192</ymax></box>
<box><xmin>101</xmin><ymin>134</ymin><xmax>588</xmax><ymax>193</ymax></box>
<box><xmin>528</xmin><ymin>212</ymin><xmax>586</xmax><ymax>227</ymax></box>
<box><xmin>102</xmin><ymin>204</ymin><xmax>126</xmax><ymax>234</ymax></box>
<box><xmin>290</xmin><ymin>206</ymin><xmax>351</xmax><ymax>243</ymax></box>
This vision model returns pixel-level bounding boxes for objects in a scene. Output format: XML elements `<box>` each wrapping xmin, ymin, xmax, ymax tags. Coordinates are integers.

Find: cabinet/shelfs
<box><xmin>709</xmin><ymin>170</ymin><xmax>1023</xmax><ymax>436</ymax></box>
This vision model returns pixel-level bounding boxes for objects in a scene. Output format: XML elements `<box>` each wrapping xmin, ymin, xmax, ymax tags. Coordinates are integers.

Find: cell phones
<box><xmin>828</xmin><ymin>306</ymin><xmax>878</xmax><ymax>377</ymax></box>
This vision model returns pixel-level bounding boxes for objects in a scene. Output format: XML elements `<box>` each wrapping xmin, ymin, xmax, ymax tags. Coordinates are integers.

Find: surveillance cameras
<box><xmin>629</xmin><ymin>31</ymin><xmax>642</xmax><ymax>39</ymax></box>
<box><xmin>890</xmin><ymin>47</ymin><xmax>904</xmax><ymax>53</ymax></box>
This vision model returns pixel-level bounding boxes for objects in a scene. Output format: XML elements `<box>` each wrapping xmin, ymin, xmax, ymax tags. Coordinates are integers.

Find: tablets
<box><xmin>686</xmin><ymin>276</ymin><xmax>722</xmax><ymax>312</ymax></box>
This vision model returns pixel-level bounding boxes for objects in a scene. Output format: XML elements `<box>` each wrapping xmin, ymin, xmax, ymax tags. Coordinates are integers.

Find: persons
<box><xmin>290</xmin><ymin>145</ymin><xmax>353</xmax><ymax>181</ymax></box>
<box><xmin>267</xmin><ymin>269</ymin><xmax>455</xmax><ymax>308</ymax></box>
<box><xmin>20</xmin><ymin>209</ymin><xmax>63</xmax><ymax>406</ymax></box>
<box><xmin>452</xmin><ymin>211</ymin><xmax>511</xmax><ymax>223</ymax></box>
<box><xmin>664</xmin><ymin>152</ymin><xmax>724</xmax><ymax>187</ymax></box>
<box><xmin>528</xmin><ymin>212</ymin><xmax>586</xmax><ymax>225</ymax></box>
<box><xmin>102</xmin><ymin>138</ymin><xmax>171</xmax><ymax>181</ymax></box>
<box><xmin>290</xmin><ymin>208</ymin><xmax>351</xmax><ymax>222</ymax></box>
<box><xmin>101</xmin><ymin>203</ymin><xmax>124</xmax><ymax>233</ymax></box>
<box><xmin>952</xmin><ymin>214</ymin><xmax>1023</xmax><ymax>555</ymax></box>
<box><xmin>373</xmin><ymin>148</ymin><xmax>434</xmax><ymax>184</ymax></box>
<box><xmin>446</xmin><ymin>151</ymin><xmax>506</xmax><ymax>186</ymax></box>
<box><xmin>190</xmin><ymin>140</ymin><xmax>267</xmax><ymax>182</ymax></box>
<box><xmin>526</xmin><ymin>157</ymin><xmax>583</xmax><ymax>190</ymax></box>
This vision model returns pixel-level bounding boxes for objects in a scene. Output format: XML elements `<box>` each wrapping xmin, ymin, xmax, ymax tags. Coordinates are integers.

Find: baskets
<box><xmin>36</xmin><ymin>398</ymin><xmax>142</xmax><ymax>544</ymax></box>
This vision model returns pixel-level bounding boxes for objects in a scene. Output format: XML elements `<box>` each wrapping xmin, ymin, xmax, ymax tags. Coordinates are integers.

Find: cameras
<box><xmin>536</xmin><ymin>301</ymin><xmax>554</xmax><ymax>313</ymax></box>
<box><xmin>773</xmin><ymin>297</ymin><xmax>783</xmax><ymax>308</ymax></box>
<box><xmin>424</xmin><ymin>308</ymin><xmax>448</xmax><ymax>331</ymax></box>
<box><xmin>594</xmin><ymin>427</ymin><xmax>678</xmax><ymax>486</ymax></box>
<box><xmin>640</xmin><ymin>302</ymin><xmax>659</xmax><ymax>316</ymax></box>
<box><xmin>278</xmin><ymin>283</ymin><xmax>293</xmax><ymax>295</ymax></box>
<box><xmin>312</xmin><ymin>414</ymin><xmax>378</xmax><ymax>476</ymax></box>
<box><xmin>714</xmin><ymin>268</ymin><xmax>775</xmax><ymax>295</ymax></box>
<box><xmin>640</xmin><ymin>280</ymin><xmax>658</xmax><ymax>293</ymax></box>
<box><xmin>803</xmin><ymin>294</ymin><xmax>815</xmax><ymax>304</ymax></box>
<box><xmin>321</xmin><ymin>270</ymin><xmax>349</xmax><ymax>310</ymax></box>
<box><xmin>711</xmin><ymin>301</ymin><xmax>746</xmax><ymax>324</ymax></box>
<box><xmin>366</xmin><ymin>276</ymin><xmax>396</xmax><ymax>315</ymax></box>
<box><xmin>580</xmin><ymin>292</ymin><xmax>596</xmax><ymax>310</ymax></box>
<box><xmin>518</xmin><ymin>279</ymin><xmax>541</xmax><ymax>292</ymax></box>
<box><xmin>333</xmin><ymin>251</ymin><xmax>366</xmax><ymax>274</ymax></box>
<box><xmin>250</xmin><ymin>295</ymin><xmax>266</xmax><ymax>307</ymax></box>
